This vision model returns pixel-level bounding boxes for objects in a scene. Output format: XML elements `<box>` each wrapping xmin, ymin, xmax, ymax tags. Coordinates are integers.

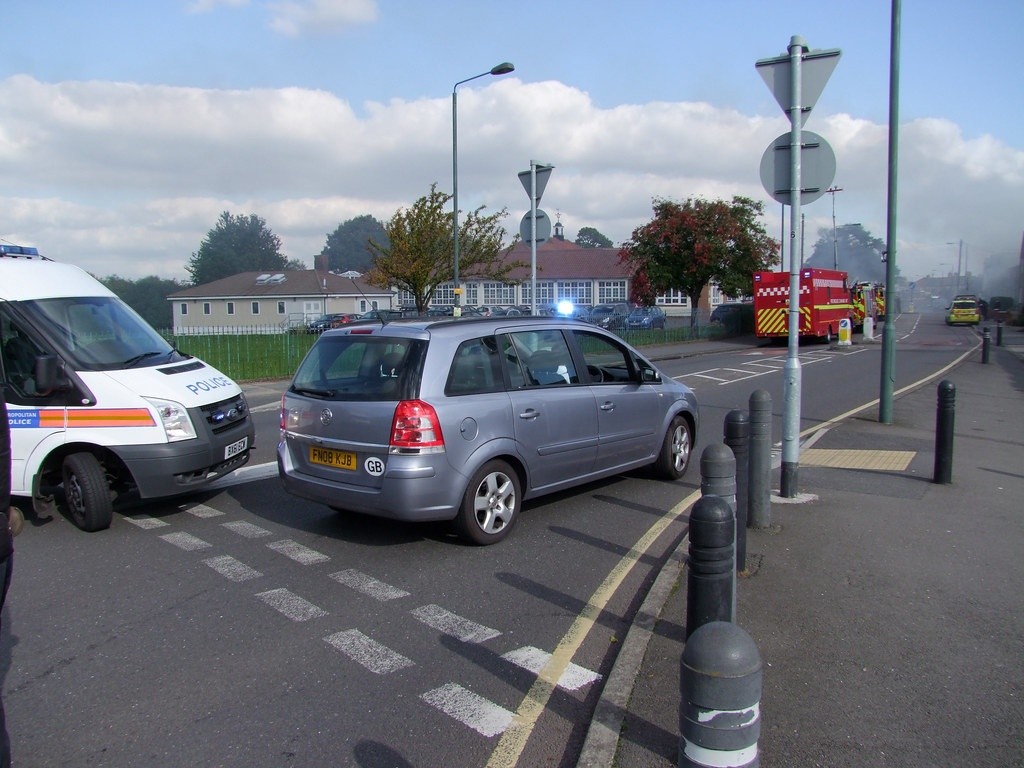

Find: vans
<box><xmin>0</xmin><ymin>243</ymin><xmax>255</xmax><ymax>532</ymax></box>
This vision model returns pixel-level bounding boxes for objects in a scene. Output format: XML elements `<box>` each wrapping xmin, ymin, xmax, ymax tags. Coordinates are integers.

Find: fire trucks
<box><xmin>873</xmin><ymin>284</ymin><xmax>886</xmax><ymax>321</ymax></box>
<box><xmin>753</xmin><ymin>268</ymin><xmax>854</xmax><ymax>344</ymax></box>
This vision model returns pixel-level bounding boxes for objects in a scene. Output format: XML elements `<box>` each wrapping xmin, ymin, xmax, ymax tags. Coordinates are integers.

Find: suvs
<box><xmin>277</xmin><ymin>315</ymin><xmax>700</xmax><ymax>546</ymax></box>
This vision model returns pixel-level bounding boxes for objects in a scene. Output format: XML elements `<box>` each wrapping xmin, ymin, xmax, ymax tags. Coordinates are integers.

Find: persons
<box><xmin>551</xmin><ymin>344</ymin><xmax>570</xmax><ymax>384</ymax></box>
<box><xmin>978</xmin><ymin>298</ymin><xmax>989</xmax><ymax>321</ymax></box>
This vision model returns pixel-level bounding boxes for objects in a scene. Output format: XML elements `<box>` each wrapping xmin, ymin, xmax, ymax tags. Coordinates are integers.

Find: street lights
<box><xmin>453</xmin><ymin>63</ymin><xmax>515</xmax><ymax>316</ymax></box>
<box><xmin>833</xmin><ymin>223</ymin><xmax>861</xmax><ymax>271</ymax></box>
<box><xmin>946</xmin><ymin>240</ymin><xmax>962</xmax><ymax>289</ymax></box>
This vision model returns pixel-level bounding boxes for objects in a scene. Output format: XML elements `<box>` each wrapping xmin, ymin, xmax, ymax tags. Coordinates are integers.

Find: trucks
<box><xmin>850</xmin><ymin>282</ymin><xmax>877</xmax><ymax>332</ymax></box>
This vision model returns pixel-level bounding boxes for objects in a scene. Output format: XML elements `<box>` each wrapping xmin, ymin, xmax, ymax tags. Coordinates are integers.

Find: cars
<box><xmin>945</xmin><ymin>300</ymin><xmax>979</xmax><ymax>326</ymax></box>
<box><xmin>624</xmin><ymin>307</ymin><xmax>666</xmax><ymax>329</ymax></box>
<box><xmin>307</xmin><ymin>306</ymin><xmax>592</xmax><ymax>325</ymax></box>
<box><xmin>954</xmin><ymin>295</ymin><xmax>984</xmax><ymax>321</ymax></box>
<box><xmin>590</xmin><ymin>303</ymin><xmax>629</xmax><ymax>330</ymax></box>
<box><xmin>711</xmin><ymin>303</ymin><xmax>754</xmax><ymax>333</ymax></box>
